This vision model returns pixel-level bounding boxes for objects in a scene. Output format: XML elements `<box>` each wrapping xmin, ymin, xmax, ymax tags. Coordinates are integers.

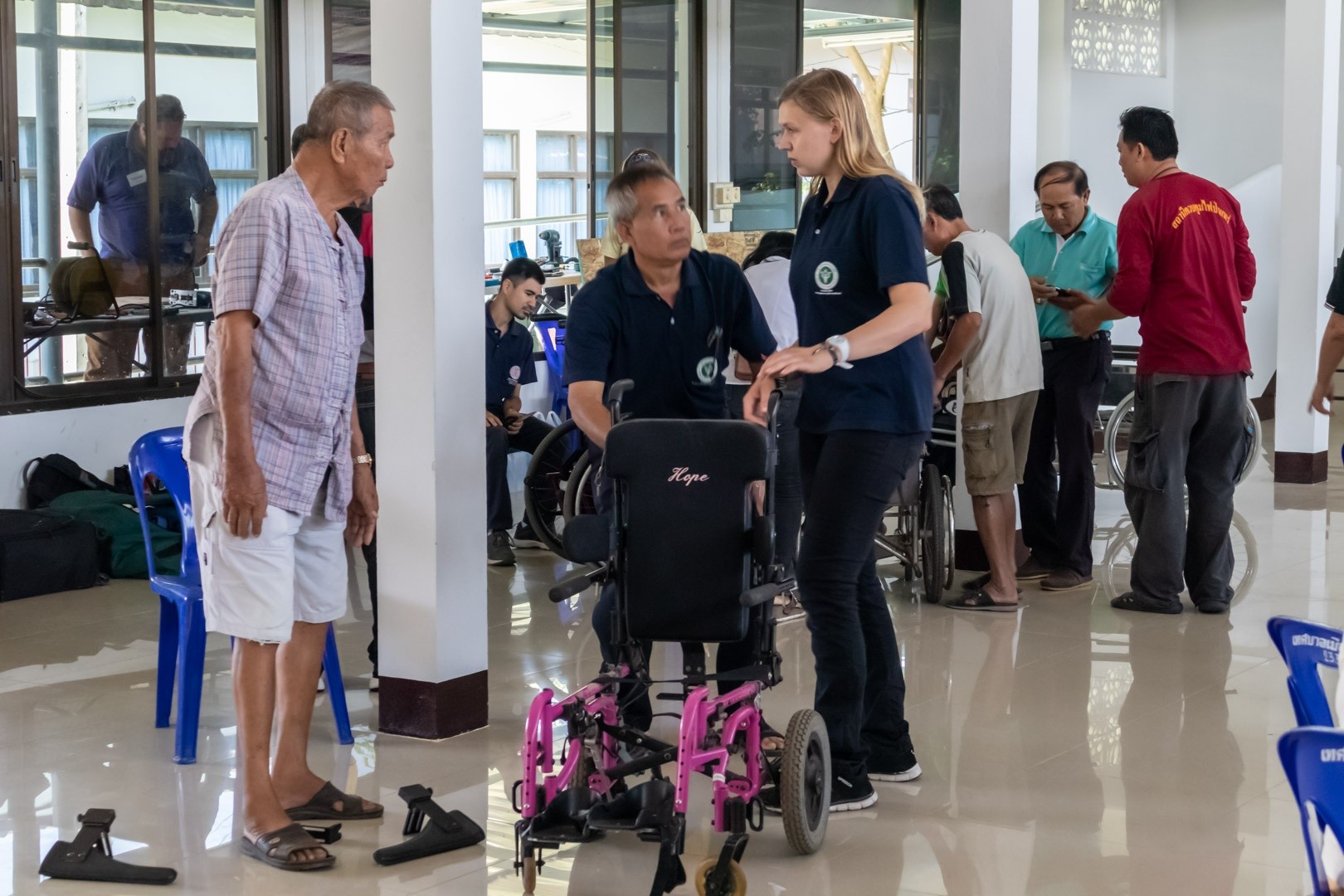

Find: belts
<box><xmin>1041</xmin><ymin>330</ymin><xmax>1111</xmax><ymax>351</ymax></box>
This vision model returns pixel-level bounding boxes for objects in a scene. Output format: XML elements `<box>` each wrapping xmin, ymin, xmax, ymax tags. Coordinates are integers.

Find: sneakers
<box><xmin>510</xmin><ymin>520</ymin><xmax>563</xmax><ymax>549</ymax></box>
<box><xmin>756</xmin><ymin>763</ymin><xmax>879</xmax><ymax>814</ymax></box>
<box><xmin>368</xmin><ymin>677</ymin><xmax>379</xmax><ymax>690</ymax></box>
<box><xmin>487</xmin><ymin>529</ymin><xmax>517</xmax><ymax>565</ymax></box>
<box><xmin>317</xmin><ymin>677</ymin><xmax>325</xmax><ymax>690</ymax></box>
<box><xmin>867</xmin><ymin>750</ymin><xmax>922</xmax><ymax>782</ymax></box>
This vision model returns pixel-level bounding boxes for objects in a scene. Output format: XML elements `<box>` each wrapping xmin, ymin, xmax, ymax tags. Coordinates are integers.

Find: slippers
<box><xmin>283</xmin><ymin>780</ymin><xmax>384</xmax><ymax>821</ymax></box>
<box><xmin>773</xmin><ymin>592</ymin><xmax>806</xmax><ymax>623</ymax></box>
<box><xmin>945</xmin><ymin>586</ymin><xmax>1018</xmax><ymax>612</ymax></box>
<box><xmin>240</xmin><ymin>823</ymin><xmax>336</xmax><ymax>871</ymax></box>
<box><xmin>962</xmin><ymin>573</ymin><xmax>1023</xmax><ymax>595</ymax></box>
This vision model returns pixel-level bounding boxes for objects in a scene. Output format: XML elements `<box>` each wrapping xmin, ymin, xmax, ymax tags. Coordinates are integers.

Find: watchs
<box><xmin>825</xmin><ymin>334</ymin><xmax>854</xmax><ymax>370</ymax></box>
<box><xmin>350</xmin><ymin>453</ymin><xmax>372</xmax><ymax>468</ymax></box>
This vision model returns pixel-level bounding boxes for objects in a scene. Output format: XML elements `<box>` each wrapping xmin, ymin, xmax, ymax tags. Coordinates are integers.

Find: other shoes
<box><xmin>1111</xmin><ymin>591</ymin><xmax>1183</xmax><ymax>614</ymax></box>
<box><xmin>1198</xmin><ymin>586</ymin><xmax>1234</xmax><ymax>613</ymax></box>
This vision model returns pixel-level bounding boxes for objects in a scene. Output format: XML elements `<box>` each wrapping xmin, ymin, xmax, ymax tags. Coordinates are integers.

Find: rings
<box><xmin>1036</xmin><ymin>298</ymin><xmax>1042</xmax><ymax>304</ymax></box>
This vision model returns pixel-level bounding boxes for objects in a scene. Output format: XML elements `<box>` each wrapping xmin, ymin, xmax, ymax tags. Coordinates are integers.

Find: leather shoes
<box><xmin>1015</xmin><ymin>554</ymin><xmax>1054</xmax><ymax>579</ymax></box>
<box><xmin>1039</xmin><ymin>568</ymin><xmax>1093</xmax><ymax>590</ymax></box>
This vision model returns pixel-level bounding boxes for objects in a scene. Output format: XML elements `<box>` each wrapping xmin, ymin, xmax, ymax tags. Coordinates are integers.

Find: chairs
<box><xmin>127</xmin><ymin>426</ymin><xmax>355</xmax><ymax>765</ymax></box>
<box><xmin>534</xmin><ymin>320</ymin><xmax>579</xmax><ymax>461</ymax></box>
<box><xmin>1267</xmin><ymin>616</ymin><xmax>1344</xmax><ymax>896</ymax></box>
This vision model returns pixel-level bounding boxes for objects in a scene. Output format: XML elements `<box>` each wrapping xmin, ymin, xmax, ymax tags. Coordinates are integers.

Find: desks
<box><xmin>24</xmin><ymin>306</ymin><xmax>215</xmax><ymax>373</ymax></box>
<box><xmin>485</xmin><ymin>269</ymin><xmax>581</xmax><ymax>333</ymax></box>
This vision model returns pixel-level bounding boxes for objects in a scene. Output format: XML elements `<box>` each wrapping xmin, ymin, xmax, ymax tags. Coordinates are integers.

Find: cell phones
<box><xmin>1045</xmin><ymin>284</ymin><xmax>1070</xmax><ymax>297</ymax></box>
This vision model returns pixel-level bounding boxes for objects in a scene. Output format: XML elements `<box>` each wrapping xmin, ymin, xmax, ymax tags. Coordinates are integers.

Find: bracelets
<box><xmin>812</xmin><ymin>341</ymin><xmax>838</xmax><ymax>366</ymax></box>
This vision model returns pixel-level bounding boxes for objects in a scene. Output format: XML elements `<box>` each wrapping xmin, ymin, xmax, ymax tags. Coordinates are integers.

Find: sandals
<box><xmin>732</xmin><ymin>710</ymin><xmax>786</xmax><ymax>757</ymax></box>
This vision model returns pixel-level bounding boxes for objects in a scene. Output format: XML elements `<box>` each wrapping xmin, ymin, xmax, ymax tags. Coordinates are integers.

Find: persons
<box><xmin>1070</xmin><ymin>106</ymin><xmax>1256</xmax><ymax>616</ymax></box>
<box><xmin>561</xmin><ymin>67</ymin><xmax>935</xmax><ymax>815</ymax></box>
<box><xmin>1306</xmin><ymin>251</ymin><xmax>1344</xmax><ymax>417</ymax></box>
<box><xmin>484</xmin><ymin>257</ymin><xmax>570</xmax><ymax>565</ymax></box>
<box><xmin>64</xmin><ymin>94</ymin><xmax>219</xmax><ymax>386</ymax></box>
<box><xmin>1007</xmin><ymin>161</ymin><xmax>1121</xmax><ymax>592</ymax></box>
<box><xmin>919</xmin><ymin>181</ymin><xmax>1045</xmax><ymax>614</ymax></box>
<box><xmin>182</xmin><ymin>80</ymin><xmax>396</xmax><ymax>872</ymax></box>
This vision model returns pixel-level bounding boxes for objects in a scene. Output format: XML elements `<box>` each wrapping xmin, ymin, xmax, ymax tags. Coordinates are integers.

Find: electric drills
<box><xmin>539</xmin><ymin>229</ymin><xmax>564</xmax><ymax>268</ymax></box>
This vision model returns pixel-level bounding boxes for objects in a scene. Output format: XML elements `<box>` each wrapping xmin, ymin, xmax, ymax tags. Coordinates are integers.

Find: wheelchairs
<box><xmin>874</xmin><ymin>311</ymin><xmax>966</xmax><ymax>603</ymax></box>
<box><xmin>511</xmin><ymin>379</ymin><xmax>830</xmax><ymax>896</ymax></box>
<box><xmin>1091</xmin><ymin>304</ymin><xmax>1262</xmax><ymax>506</ymax></box>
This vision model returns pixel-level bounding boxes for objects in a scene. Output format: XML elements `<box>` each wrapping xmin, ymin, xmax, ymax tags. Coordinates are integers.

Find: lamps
<box><xmin>822</xmin><ymin>30</ymin><xmax>913</xmax><ymax>49</ymax></box>
